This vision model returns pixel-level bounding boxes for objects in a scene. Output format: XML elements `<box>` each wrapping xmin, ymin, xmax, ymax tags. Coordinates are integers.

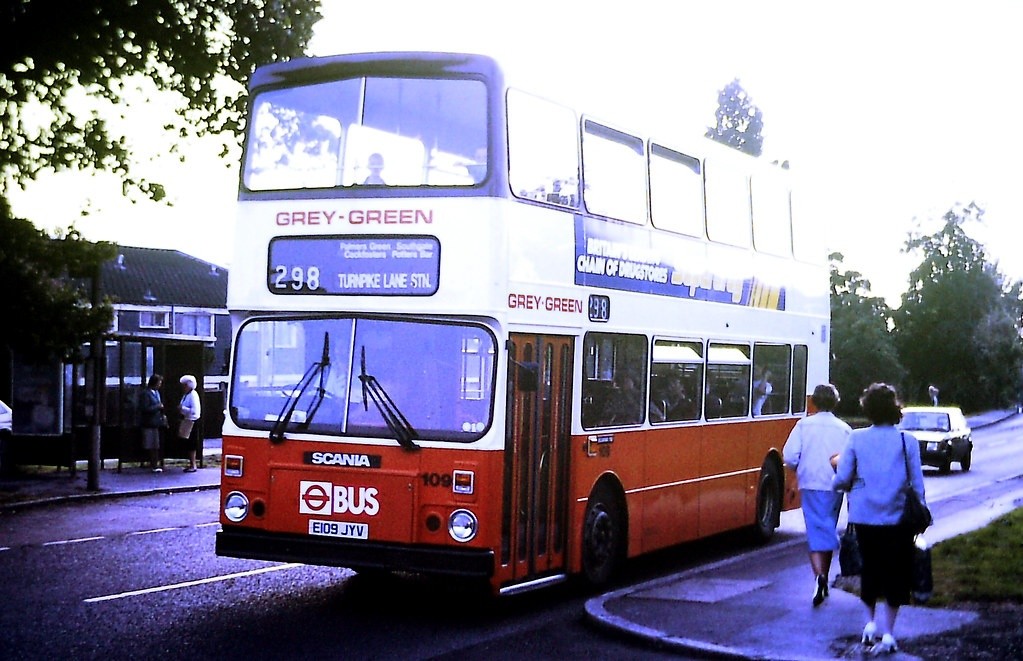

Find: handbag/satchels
<box><xmin>839</xmin><ymin>521</ymin><xmax>862</xmax><ymax>578</ymax></box>
<box><xmin>909</xmin><ymin>537</ymin><xmax>934</xmax><ymax>606</ymax></box>
<box><xmin>142</xmin><ymin>392</ymin><xmax>161</xmax><ymax>427</ymax></box>
<box><xmin>179</xmin><ymin>408</ymin><xmax>195</xmax><ymax>439</ymax></box>
<box><xmin>904</xmin><ymin>488</ymin><xmax>930</xmax><ymax>534</ymax></box>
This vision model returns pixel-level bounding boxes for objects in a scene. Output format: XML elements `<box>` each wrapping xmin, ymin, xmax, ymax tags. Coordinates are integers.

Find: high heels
<box><xmin>812</xmin><ymin>576</ymin><xmax>828</xmax><ymax>606</ymax></box>
<box><xmin>861</xmin><ymin>621</ymin><xmax>878</xmax><ymax>646</ymax></box>
<box><xmin>881</xmin><ymin>634</ymin><xmax>899</xmax><ymax>652</ymax></box>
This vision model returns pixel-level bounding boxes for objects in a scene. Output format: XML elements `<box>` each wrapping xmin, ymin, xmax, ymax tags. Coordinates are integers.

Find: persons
<box><xmin>178</xmin><ymin>375</ymin><xmax>201</xmax><ymax>472</ymax></box>
<box><xmin>519</xmin><ymin>179</ymin><xmax>561</xmax><ymax>204</ymax></box>
<box><xmin>582</xmin><ymin>356</ymin><xmax>789</xmax><ymax>428</ymax></box>
<box><xmin>363</xmin><ymin>152</ymin><xmax>387</xmax><ymax>185</ymax></box>
<box><xmin>781</xmin><ymin>385</ymin><xmax>853</xmax><ymax>607</ymax></box>
<box><xmin>832</xmin><ymin>383</ymin><xmax>932</xmax><ymax>655</ymax></box>
<box><xmin>139</xmin><ymin>374</ymin><xmax>165</xmax><ymax>471</ymax></box>
<box><xmin>306</xmin><ymin>332</ymin><xmax>370</xmax><ymax>402</ymax></box>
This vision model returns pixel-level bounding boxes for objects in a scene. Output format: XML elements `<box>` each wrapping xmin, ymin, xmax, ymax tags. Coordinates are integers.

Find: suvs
<box><xmin>894</xmin><ymin>406</ymin><xmax>973</xmax><ymax>477</ymax></box>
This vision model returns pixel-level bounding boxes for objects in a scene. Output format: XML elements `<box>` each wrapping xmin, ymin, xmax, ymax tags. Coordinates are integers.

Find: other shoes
<box><xmin>183</xmin><ymin>467</ymin><xmax>197</xmax><ymax>472</ymax></box>
<box><xmin>150</xmin><ymin>465</ymin><xmax>163</xmax><ymax>473</ymax></box>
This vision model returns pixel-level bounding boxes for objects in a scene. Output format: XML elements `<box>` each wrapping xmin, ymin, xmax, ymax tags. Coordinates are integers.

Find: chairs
<box><xmin>651</xmin><ymin>391</ymin><xmax>774</xmax><ymax>423</ymax></box>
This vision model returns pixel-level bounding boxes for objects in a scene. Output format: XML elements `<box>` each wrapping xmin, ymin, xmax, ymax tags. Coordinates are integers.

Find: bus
<box><xmin>214</xmin><ymin>48</ymin><xmax>829</xmax><ymax>598</ymax></box>
